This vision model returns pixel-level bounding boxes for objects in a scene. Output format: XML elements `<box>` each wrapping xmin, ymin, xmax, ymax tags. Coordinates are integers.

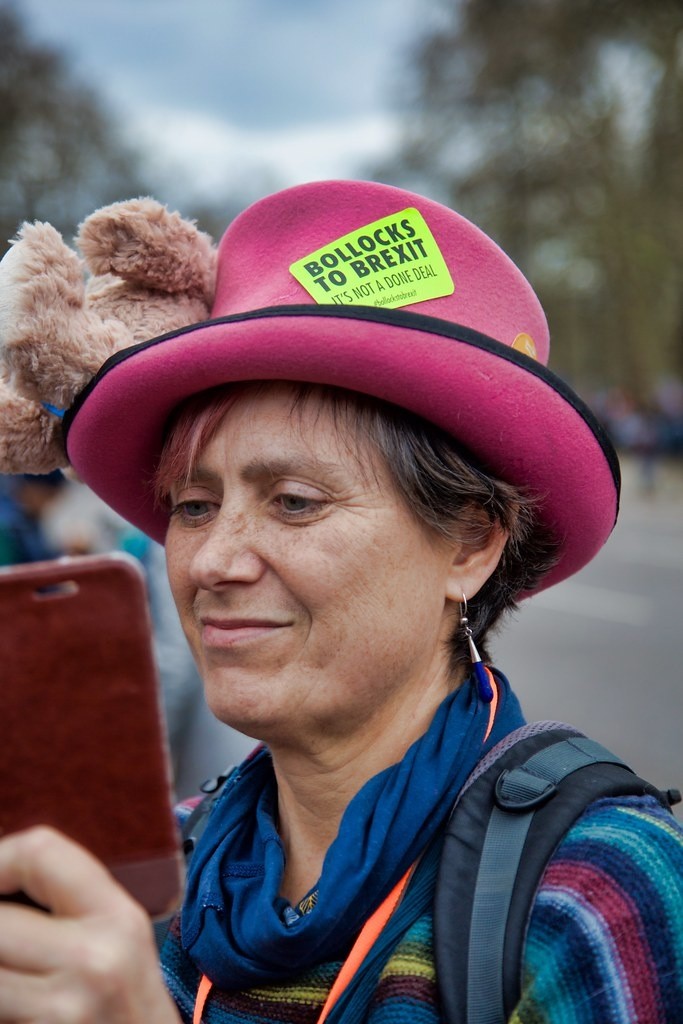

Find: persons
<box><xmin>0</xmin><ymin>179</ymin><xmax>683</xmax><ymax>1024</ymax></box>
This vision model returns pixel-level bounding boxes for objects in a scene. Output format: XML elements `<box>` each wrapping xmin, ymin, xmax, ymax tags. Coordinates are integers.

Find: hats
<box><xmin>62</xmin><ymin>175</ymin><xmax>622</xmax><ymax>606</ymax></box>
